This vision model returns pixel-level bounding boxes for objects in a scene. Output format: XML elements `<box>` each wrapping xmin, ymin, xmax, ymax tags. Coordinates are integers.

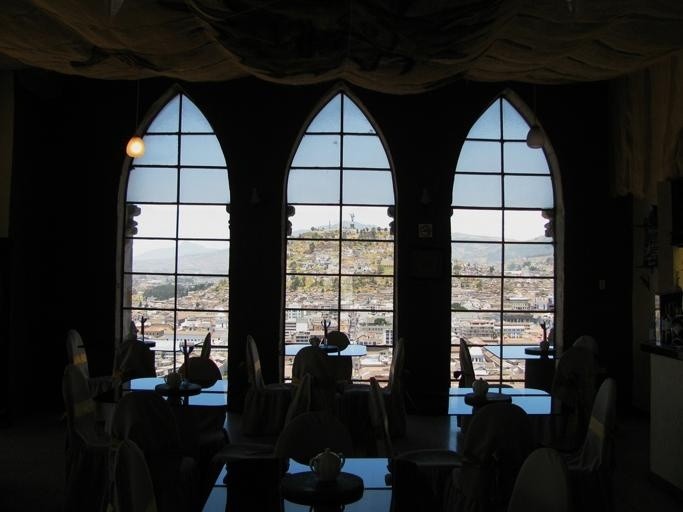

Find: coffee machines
<box><xmin>653</xmin><ymin>289</ymin><xmax>681</xmax><ymax>348</ymax></box>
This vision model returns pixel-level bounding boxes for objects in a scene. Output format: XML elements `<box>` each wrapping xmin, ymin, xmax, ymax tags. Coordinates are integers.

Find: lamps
<box><xmin>126</xmin><ymin>83</ymin><xmax>146</xmax><ymax>159</ymax></box>
<box><xmin>526</xmin><ymin>84</ymin><xmax>547</xmax><ymax>151</ymax></box>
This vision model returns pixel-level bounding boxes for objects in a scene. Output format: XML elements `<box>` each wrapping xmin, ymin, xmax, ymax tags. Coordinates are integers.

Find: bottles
<box><xmin>309</xmin><ymin>447</ymin><xmax>345</xmax><ymax>481</ymax></box>
<box><xmin>472</xmin><ymin>377</ymin><xmax>489</xmax><ymax>397</ymax></box>
<box><xmin>164</xmin><ymin>373</ymin><xmax>184</xmax><ymax>388</ymax></box>
